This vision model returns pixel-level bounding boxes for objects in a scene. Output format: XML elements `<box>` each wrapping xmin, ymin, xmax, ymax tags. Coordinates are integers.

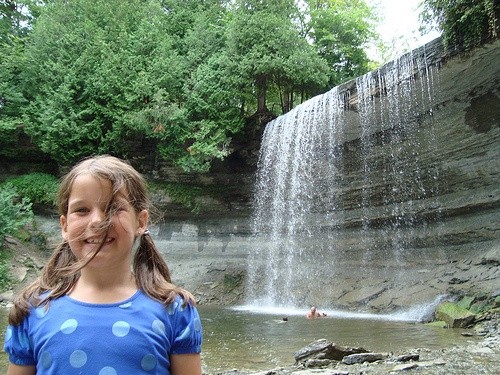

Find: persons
<box><xmin>4</xmin><ymin>154</ymin><xmax>203</xmax><ymax>375</ymax></box>
<box><xmin>307</xmin><ymin>306</ymin><xmax>327</xmax><ymax>318</ymax></box>
